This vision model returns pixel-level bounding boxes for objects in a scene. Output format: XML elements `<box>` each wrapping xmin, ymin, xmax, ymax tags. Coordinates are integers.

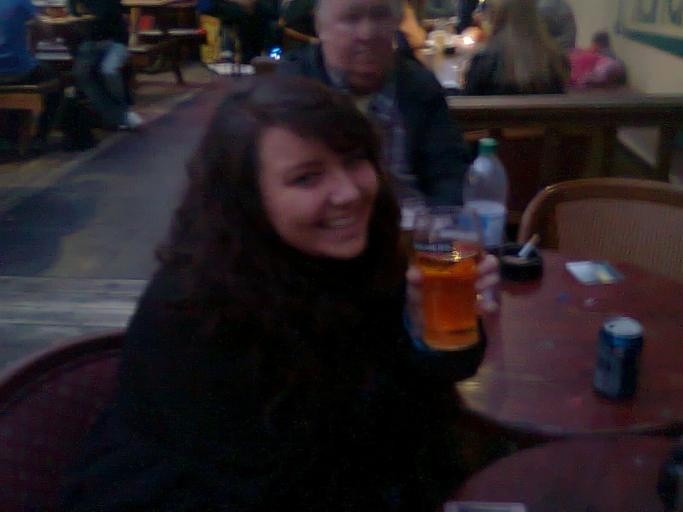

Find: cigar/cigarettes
<box><xmin>519</xmin><ymin>233</ymin><xmax>541</xmax><ymax>259</ymax></box>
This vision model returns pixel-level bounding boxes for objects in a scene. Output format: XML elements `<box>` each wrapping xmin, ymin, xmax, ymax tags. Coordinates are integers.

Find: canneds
<box><xmin>593</xmin><ymin>316</ymin><xmax>644</xmax><ymax>401</ymax></box>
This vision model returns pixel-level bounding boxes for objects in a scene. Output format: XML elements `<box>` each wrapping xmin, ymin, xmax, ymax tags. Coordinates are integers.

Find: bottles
<box><xmin>465</xmin><ymin>137</ymin><xmax>509</xmax><ymax>259</ymax></box>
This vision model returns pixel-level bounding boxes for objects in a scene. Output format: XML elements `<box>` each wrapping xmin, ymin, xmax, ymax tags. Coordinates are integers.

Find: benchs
<box><xmin>0</xmin><ymin>25</ymin><xmax>208</xmax><ymax>157</ymax></box>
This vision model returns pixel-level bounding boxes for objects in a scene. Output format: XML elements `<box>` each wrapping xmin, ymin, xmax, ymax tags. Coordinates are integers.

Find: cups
<box><xmin>413</xmin><ymin>204</ymin><xmax>487</xmax><ymax>350</ymax></box>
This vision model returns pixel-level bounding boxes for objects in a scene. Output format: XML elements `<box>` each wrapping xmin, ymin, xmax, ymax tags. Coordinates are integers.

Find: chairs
<box><xmin>517</xmin><ymin>177</ymin><xmax>683</xmax><ymax>281</ymax></box>
<box><xmin>0</xmin><ymin>326</ymin><xmax>130</xmax><ymax>511</ymax></box>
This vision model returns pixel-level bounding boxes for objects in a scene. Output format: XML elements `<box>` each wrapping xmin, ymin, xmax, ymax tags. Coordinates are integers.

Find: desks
<box><xmin>445</xmin><ymin>91</ymin><xmax>683</xmax><ymax>182</ymax></box>
<box><xmin>439</xmin><ymin>248</ymin><xmax>683</xmax><ymax>512</ymax></box>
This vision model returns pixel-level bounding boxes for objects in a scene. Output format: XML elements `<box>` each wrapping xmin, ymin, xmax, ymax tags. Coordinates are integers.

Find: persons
<box><xmin>2</xmin><ymin>2</ymin><xmax>129</xmax><ymax>146</ymax></box>
<box><xmin>59</xmin><ymin>76</ymin><xmax>500</xmax><ymax>512</ymax></box>
<box><xmin>274</xmin><ymin>0</ymin><xmax>474</xmax><ymax>229</ymax></box>
<box><xmin>392</xmin><ymin>0</ymin><xmax>575</xmax><ymax>94</ymax></box>
<box><xmin>198</xmin><ymin>0</ymin><xmax>321</xmax><ymax>64</ymax></box>
